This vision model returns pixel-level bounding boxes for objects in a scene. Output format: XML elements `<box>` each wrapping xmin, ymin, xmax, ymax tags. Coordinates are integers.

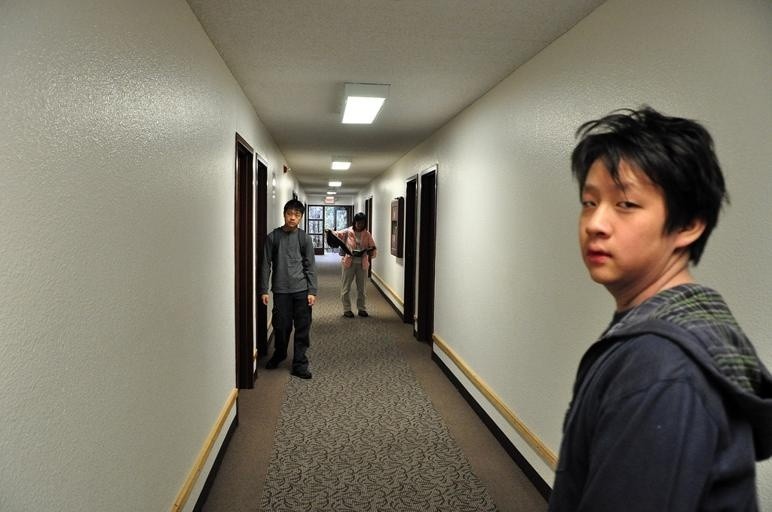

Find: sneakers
<box><xmin>265</xmin><ymin>349</ymin><xmax>314</xmax><ymax>379</ymax></box>
<box><xmin>343</xmin><ymin>310</ymin><xmax>369</xmax><ymax>318</ymax></box>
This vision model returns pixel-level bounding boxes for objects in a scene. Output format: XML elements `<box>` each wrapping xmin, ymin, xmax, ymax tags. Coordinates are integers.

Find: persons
<box><xmin>325</xmin><ymin>211</ymin><xmax>378</xmax><ymax>317</ymax></box>
<box><xmin>547</xmin><ymin>102</ymin><xmax>772</xmax><ymax>510</ymax></box>
<box><xmin>259</xmin><ymin>200</ymin><xmax>319</xmax><ymax>380</ymax></box>
